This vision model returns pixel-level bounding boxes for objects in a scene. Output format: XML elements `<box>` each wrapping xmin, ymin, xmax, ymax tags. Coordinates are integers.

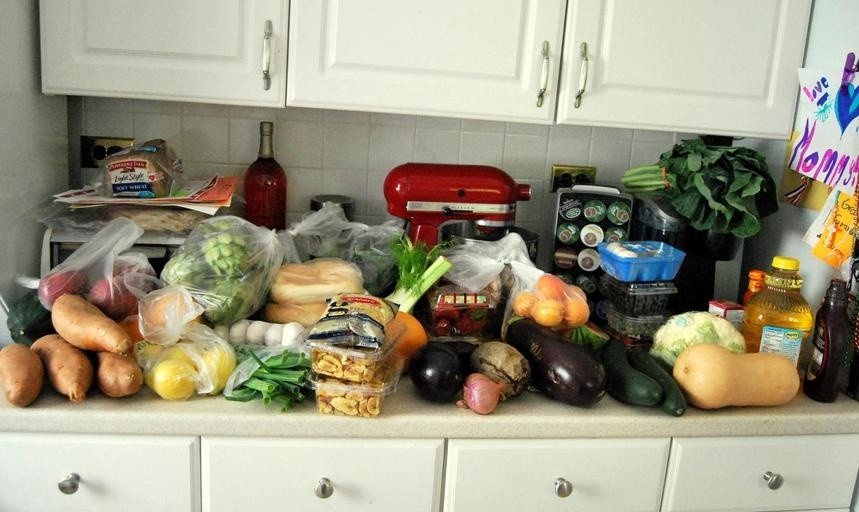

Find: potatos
<box><xmin>96</xmin><ymin>351</ymin><xmax>144</xmax><ymax>398</ymax></box>
<box><xmin>0</xmin><ymin>343</ymin><xmax>44</xmax><ymax>408</ymax></box>
<box><xmin>51</xmin><ymin>294</ymin><xmax>134</xmax><ymax>354</ymax></box>
<box><xmin>30</xmin><ymin>334</ymin><xmax>95</xmax><ymax>403</ymax></box>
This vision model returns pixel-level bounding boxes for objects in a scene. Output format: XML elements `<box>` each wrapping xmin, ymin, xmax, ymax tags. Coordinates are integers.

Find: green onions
<box><xmin>224</xmin><ymin>348</ymin><xmax>314</xmax><ymax>412</ymax></box>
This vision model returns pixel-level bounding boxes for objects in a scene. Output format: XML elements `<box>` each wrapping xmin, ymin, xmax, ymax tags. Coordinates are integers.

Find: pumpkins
<box><xmin>673</xmin><ymin>343</ymin><xmax>800</xmax><ymax>410</ymax></box>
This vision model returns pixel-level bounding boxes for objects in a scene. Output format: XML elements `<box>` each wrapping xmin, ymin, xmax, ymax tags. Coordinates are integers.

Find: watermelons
<box><xmin>559</xmin><ymin>320</ymin><xmax>611</xmax><ymax>353</ymax></box>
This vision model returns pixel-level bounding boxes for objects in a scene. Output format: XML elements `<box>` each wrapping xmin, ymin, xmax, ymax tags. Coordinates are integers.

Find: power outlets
<box><xmin>77</xmin><ymin>134</ymin><xmax>136</xmax><ymax>170</ymax></box>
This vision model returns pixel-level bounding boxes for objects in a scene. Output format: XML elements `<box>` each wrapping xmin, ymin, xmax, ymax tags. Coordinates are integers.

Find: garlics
<box><xmin>214</xmin><ymin>318</ymin><xmax>306</xmax><ymax>346</ymax></box>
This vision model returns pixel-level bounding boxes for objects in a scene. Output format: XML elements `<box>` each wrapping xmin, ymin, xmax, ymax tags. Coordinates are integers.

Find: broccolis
<box><xmin>651</xmin><ymin>311</ymin><xmax>746</xmax><ymax>366</ymax></box>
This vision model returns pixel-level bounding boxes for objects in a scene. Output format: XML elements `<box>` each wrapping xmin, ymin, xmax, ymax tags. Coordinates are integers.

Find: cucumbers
<box><xmin>627</xmin><ymin>348</ymin><xmax>688</xmax><ymax>417</ymax></box>
<box><xmin>597</xmin><ymin>339</ymin><xmax>662</xmax><ymax>418</ymax></box>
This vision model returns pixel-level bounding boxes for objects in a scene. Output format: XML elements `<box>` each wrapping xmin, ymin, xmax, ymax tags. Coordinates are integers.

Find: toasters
<box><xmin>37</xmin><ymin>226</ymin><xmax>185</xmax><ymax>286</ymax></box>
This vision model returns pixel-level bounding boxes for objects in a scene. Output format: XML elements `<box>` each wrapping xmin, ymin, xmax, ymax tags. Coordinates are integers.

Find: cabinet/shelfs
<box><xmin>288</xmin><ymin>0</ymin><xmax>816</xmax><ymax>143</ymax></box>
<box><xmin>443</xmin><ymin>434</ymin><xmax>673</xmax><ymax>512</ymax></box>
<box><xmin>33</xmin><ymin>0</ymin><xmax>290</xmax><ymax>113</ymax></box>
<box><xmin>659</xmin><ymin>432</ymin><xmax>859</xmax><ymax>512</ymax></box>
<box><xmin>0</xmin><ymin>432</ymin><xmax>198</xmax><ymax>512</ymax></box>
<box><xmin>200</xmin><ymin>439</ymin><xmax>445</xmax><ymax>512</ymax></box>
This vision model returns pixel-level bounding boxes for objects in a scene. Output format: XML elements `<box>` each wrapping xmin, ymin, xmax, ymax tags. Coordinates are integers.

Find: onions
<box><xmin>462</xmin><ymin>373</ymin><xmax>501</xmax><ymax>415</ymax></box>
<box><xmin>38</xmin><ymin>262</ymin><xmax>150</xmax><ymax>317</ymax></box>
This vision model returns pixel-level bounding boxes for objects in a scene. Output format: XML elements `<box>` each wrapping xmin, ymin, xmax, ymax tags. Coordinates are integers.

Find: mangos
<box><xmin>150</xmin><ymin>346</ymin><xmax>235</xmax><ymax>399</ymax></box>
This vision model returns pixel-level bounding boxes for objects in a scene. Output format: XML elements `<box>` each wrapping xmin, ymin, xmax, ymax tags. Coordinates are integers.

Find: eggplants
<box><xmin>502</xmin><ymin>318</ymin><xmax>608</xmax><ymax>406</ymax></box>
<box><xmin>410</xmin><ymin>341</ymin><xmax>477</xmax><ymax>403</ymax></box>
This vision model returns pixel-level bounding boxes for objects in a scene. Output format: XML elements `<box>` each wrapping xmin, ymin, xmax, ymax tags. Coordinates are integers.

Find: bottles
<box><xmin>242</xmin><ymin>121</ymin><xmax>287</xmax><ymax>229</ymax></box>
<box><xmin>307</xmin><ymin>195</ymin><xmax>354</xmax><ymax>262</ymax></box>
<box><xmin>740</xmin><ymin>269</ymin><xmax>766</xmax><ymax>302</ymax></box>
<box><xmin>802</xmin><ymin>278</ymin><xmax>852</xmax><ymax>404</ymax></box>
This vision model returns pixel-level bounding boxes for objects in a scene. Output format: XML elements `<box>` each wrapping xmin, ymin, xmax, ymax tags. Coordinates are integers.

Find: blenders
<box><xmin>383</xmin><ymin>162</ymin><xmax>541</xmax><ymax>344</ymax></box>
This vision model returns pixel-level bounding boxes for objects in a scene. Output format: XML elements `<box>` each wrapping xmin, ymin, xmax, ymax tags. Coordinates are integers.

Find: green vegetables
<box><xmin>621</xmin><ymin>138</ymin><xmax>779</xmax><ymax>240</ymax></box>
<box><xmin>386</xmin><ymin>254</ymin><xmax>452</xmax><ymax>313</ymax></box>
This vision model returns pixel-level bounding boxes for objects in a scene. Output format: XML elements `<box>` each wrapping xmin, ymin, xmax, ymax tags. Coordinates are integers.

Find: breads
<box><xmin>264</xmin><ymin>260</ymin><xmax>363</xmax><ymax>328</ymax></box>
<box><xmin>104</xmin><ymin>139</ymin><xmax>177</xmax><ymax>198</ymax></box>
